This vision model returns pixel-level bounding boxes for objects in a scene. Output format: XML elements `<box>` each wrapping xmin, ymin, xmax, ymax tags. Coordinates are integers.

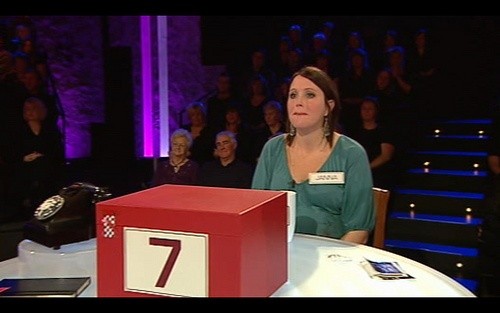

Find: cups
<box><xmin>279</xmin><ymin>191</ymin><xmax>297</xmax><ymax>244</ymax></box>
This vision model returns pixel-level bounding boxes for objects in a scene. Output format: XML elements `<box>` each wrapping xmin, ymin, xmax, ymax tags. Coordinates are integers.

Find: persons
<box><xmin>252</xmin><ymin>67</ymin><xmax>376</xmax><ymax>245</ymax></box>
<box><xmin>0</xmin><ymin>15</ymin><xmax>63</xmax><ymax>223</ymax></box>
<box><xmin>149</xmin><ymin>23</ymin><xmax>437</xmax><ymax>211</ymax></box>
<box><xmin>487</xmin><ymin>113</ymin><xmax>500</xmax><ymax>174</ymax></box>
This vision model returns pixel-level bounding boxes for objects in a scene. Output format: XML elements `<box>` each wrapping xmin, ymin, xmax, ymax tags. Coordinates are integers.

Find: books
<box><xmin>0</xmin><ymin>277</ymin><xmax>91</xmax><ymax>298</ymax></box>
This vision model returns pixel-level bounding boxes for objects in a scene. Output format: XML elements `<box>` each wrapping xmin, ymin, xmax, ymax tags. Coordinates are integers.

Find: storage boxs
<box><xmin>97</xmin><ymin>184</ymin><xmax>289</xmax><ymax>297</ymax></box>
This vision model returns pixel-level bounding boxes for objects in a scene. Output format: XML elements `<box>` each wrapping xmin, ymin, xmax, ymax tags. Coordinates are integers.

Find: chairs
<box><xmin>371</xmin><ymin>187</ymin><xmax>389</xmax><ymax>247</ymax></box>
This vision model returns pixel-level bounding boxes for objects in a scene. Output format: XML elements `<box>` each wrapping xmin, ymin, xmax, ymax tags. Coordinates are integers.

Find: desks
<box><xmin>0</xmin><ymin>234</ymin><xmax>477</xmax><ymax>297</ymax></box>
